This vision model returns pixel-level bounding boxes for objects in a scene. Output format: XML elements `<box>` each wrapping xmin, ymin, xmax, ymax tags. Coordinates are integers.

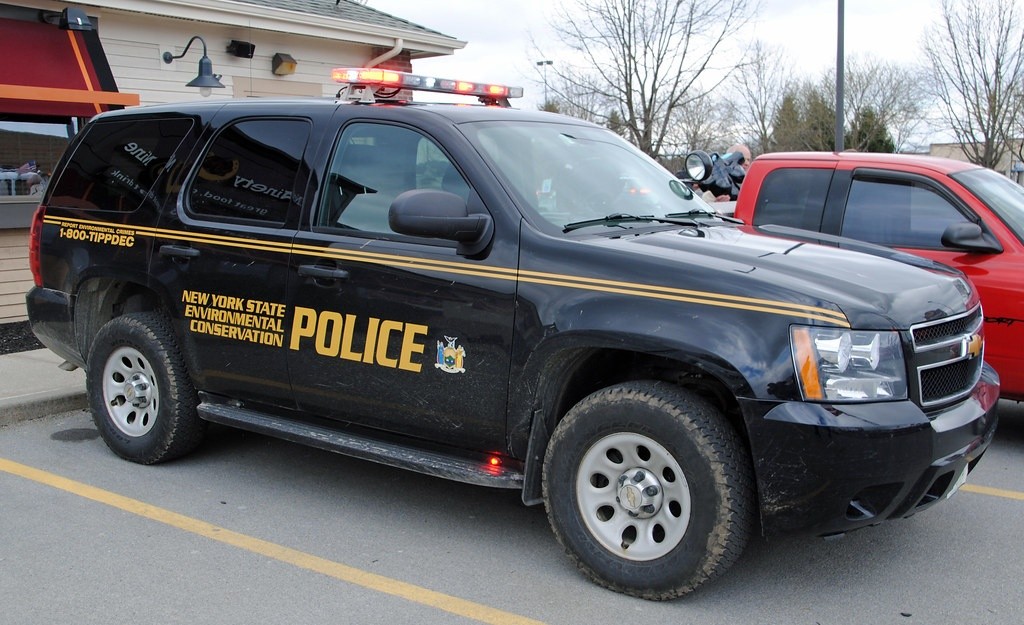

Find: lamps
<box><xmin>272</xmin><ymin>52</ymin><xmax>298</xmax><ymax>76</ymax></box>
<box><xmin>40</xmin><ymin>6</ymin><xmax>93</xmax><ymax>32</ymax></box>
<box><xmin>163</xmin><ymin>36</ymin><xmax>226</xmax><ymax>97</ymax></box>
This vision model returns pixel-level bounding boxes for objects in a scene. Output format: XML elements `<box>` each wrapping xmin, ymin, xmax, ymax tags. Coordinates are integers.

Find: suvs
<box><xmin>23</xmin><ymin>70</ymin><xmax>1001</xmax><ymax>604</ymax></box>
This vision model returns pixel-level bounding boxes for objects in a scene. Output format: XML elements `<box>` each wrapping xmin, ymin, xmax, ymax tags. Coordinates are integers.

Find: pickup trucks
<box><xmin>702</xmin><ymin>152</ymin><xmax>1024</xmax><ymax>403</ymax></box>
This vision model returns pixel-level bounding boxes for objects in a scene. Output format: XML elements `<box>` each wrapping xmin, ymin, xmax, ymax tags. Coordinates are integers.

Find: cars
<box><xmin>0</xmin><ymin>172</ymin><xmax>30</xmax><ymax>196</ymax></box>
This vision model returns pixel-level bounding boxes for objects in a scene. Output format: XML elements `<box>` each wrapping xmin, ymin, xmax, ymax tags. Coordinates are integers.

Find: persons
<box><xmin>692</xmin><ymin>183</ymin><xmax>703</xmax><ymax>199</ymax></box>
<box><xmin>701</xmin><ymin>144</ymin><xmax>751</xmax><ymax>205</ymax></box>
